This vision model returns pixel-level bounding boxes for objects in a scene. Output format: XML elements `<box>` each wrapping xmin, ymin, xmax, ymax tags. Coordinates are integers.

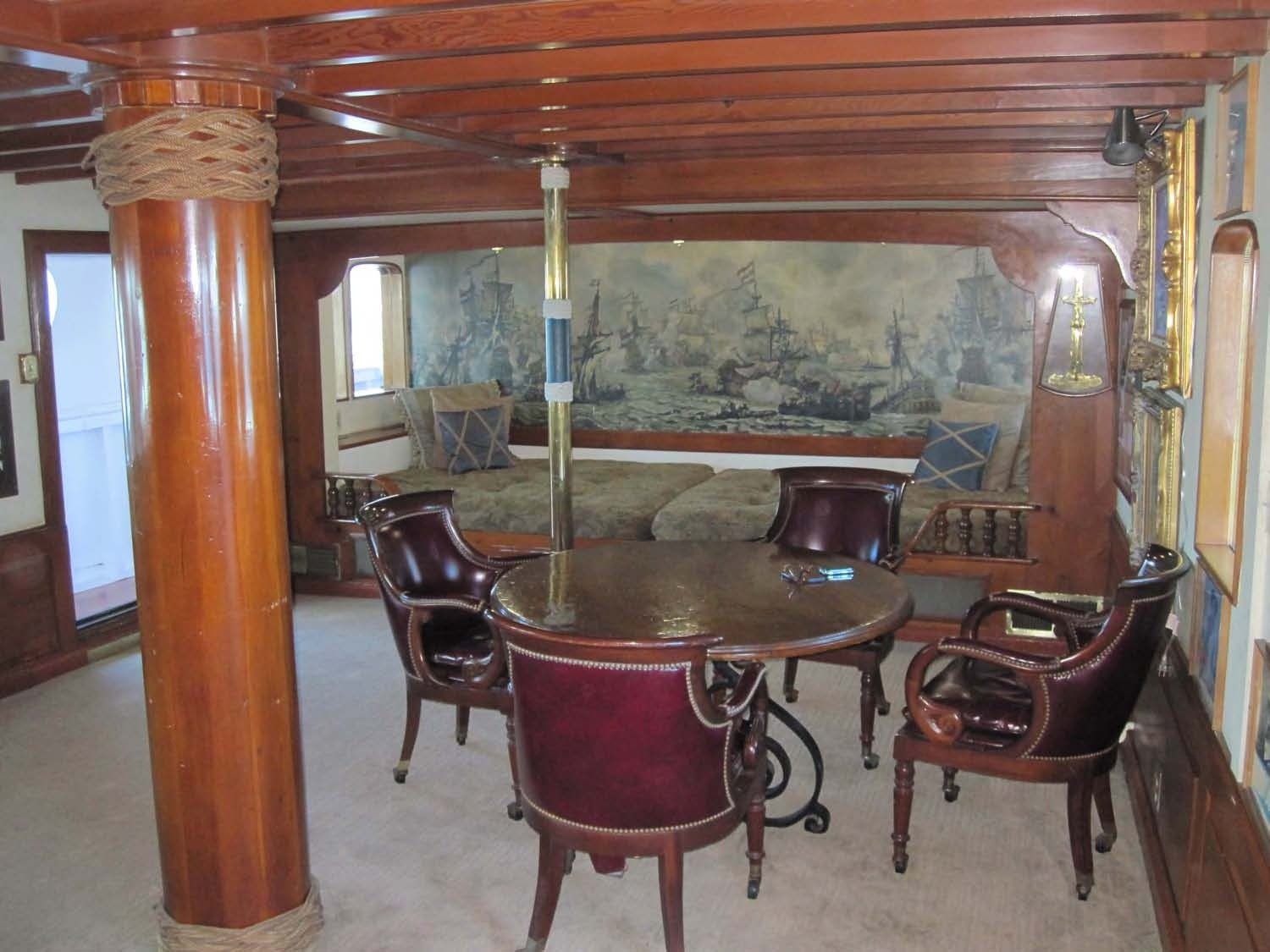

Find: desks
<box><xmin>494</xmin><ymin>539</ymin><xmax>914</xmax><ymax>836</ymax></box>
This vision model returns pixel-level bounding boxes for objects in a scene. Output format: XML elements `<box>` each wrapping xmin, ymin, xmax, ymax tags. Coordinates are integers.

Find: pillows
<box><xmin>423</xmin><ymin>388</ymin><xmax>521</xmax><ymax>474</ymax></box>
<box><xmin>933</xmin><ymin>395</ymin><xmax>1031</xmax><ymax>489</ymax></box>
<box><xmin>960</xmin><ymin>381</ymin><xmax>1040</xmax><ymax>487</ymax></box>
<box><xmin>912</xmin><ymin>419</ymin><xmax>1003</xmax><ymax>489</ymax></box>
<box><xmin>391</xmin><ymin>375</ymin><xmax>503</xmax><ymax>472</ymax></box>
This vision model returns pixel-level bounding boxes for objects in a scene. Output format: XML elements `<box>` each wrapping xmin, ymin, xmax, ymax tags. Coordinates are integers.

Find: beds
<box><xmin>372</xmin><ymin>458</ymin><xmax>714</xmax><ymax>541</ymax></box>
<box><xmin>651</xmin><ymin>467</ymin><xmax>1040</xmax><ymax>570</ymax></box>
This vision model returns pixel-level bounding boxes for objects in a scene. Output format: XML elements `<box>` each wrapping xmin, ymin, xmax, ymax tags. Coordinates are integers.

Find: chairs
<box><xmin>480</xmin><ymin>608</ymin><xmax>774</xmax><ymax>952</ymax></box>
<box><xmin>743</xmin><ymin>463</ymin><xmax>912</xmax><ymax>768</ymax></box>
<box><xmin>891</xmin><ymin>540</ymin><xmax>1191</xmax><ymax>900</ymax></box>
<box><xmin>355</xmin><ymin>489</ymin><xmax>549</xmax><ymax>821</ymax></box>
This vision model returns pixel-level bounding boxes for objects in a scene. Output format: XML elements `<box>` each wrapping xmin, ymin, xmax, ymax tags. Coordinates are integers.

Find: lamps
<box><xmin>1103</xmin><ymin>105</ymin><xmax>1184</xmax><ymax>171</ymax></box>
